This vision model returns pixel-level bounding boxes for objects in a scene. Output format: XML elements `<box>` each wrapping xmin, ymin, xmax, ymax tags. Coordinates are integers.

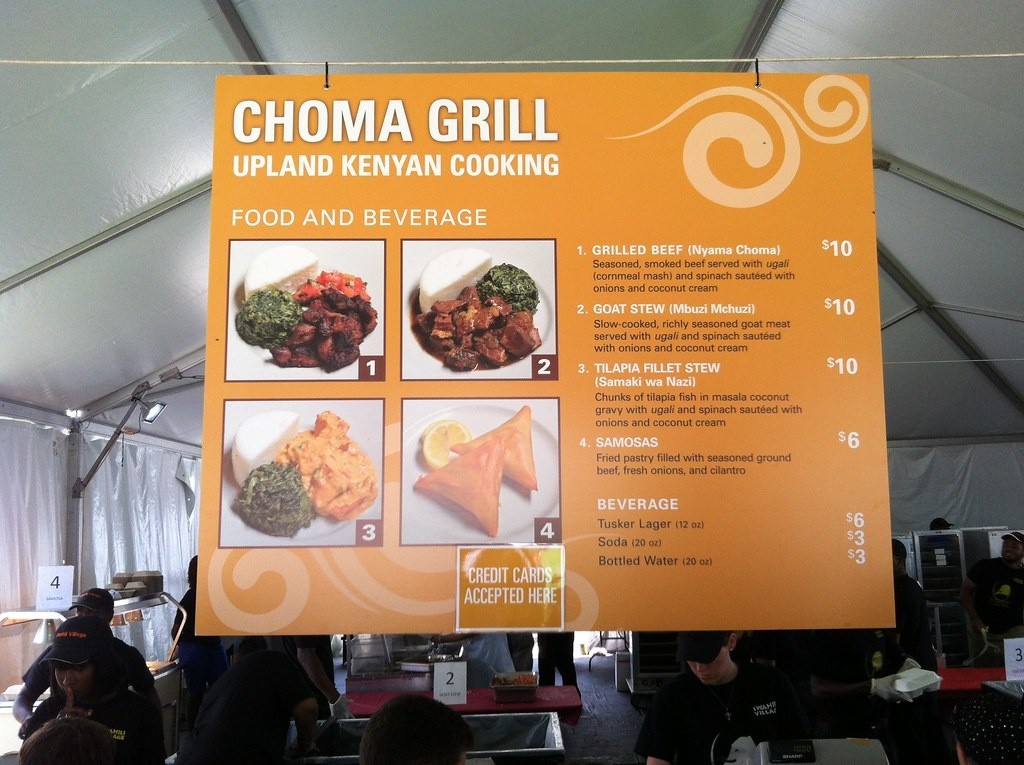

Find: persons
<box><xmin>507</xmin><ymin>633</ymin><xmax>534</xmax><ymax>671</ymax></box>
<box><xmin>14</xmin><ymin>587</ymin><xmax>158</xmax><ymax>724</ymax></box>
<box><xmin>264</xmin><ymin>634</ymin><xmax>355</xmax><ymax>721</ymax></box>
<box><xmin>633</xmin><ymin>630</ymin><xmax>812</xmax><ymax>765</ymax></box>
<box><xmin>359</xmin><ymin>694</ymin><xmax>474</xmax><ymax>765</ymax></box>
<box><xmin>17</xmin><ymin>717</ymin><xmax>117</xmax><ymax>765</ymax></box>
<box><xmin>537</xmin><ymin>632</ymin><xmax>582</xmax><ymax>717</ymax></box>
<box><xmin>930</xmin><ymin>518</ymin><xmax>954</xmax><ymax>531</ymax></box>
<box><xmin>959</xmin><ymin>532</ymin><xmax>1024</xmax><ymax>667</ymax></box>
<box><xmin>747</xmin><ymin>539</ymin><xmax>952</xmax><ymax>765</ymax></box>
<box><xmin>174</xmin><ymin>648</ymin><xmax>319</xmax><ymax>765</ymax></box>
<box><xmin>951</xmin><ymin>692</ymin><xmax>1024</xmax><ymax>765</ymax></box>
<box><xmin>170</xmin><ymin>555</ymin><xmax>229</xmax><ymax>728</ymax></box>
<box><xmin>24</xmin><ymin>615</ymin><xmax>168</xmax><ymax>765</ymax></box>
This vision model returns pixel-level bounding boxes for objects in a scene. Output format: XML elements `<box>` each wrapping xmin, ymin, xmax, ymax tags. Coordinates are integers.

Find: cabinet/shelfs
<box><xmin>0</xmin><ymin>597</ymin><xmax>179</xmax><ymax>765</ymax></box>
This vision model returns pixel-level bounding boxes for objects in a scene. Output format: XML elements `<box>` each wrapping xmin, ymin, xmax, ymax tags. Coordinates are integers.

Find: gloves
<box><xmin>871</xmin><ymin>674</ymin><xmax>913</xmax><ymax>703</ymax></box>
<box><xmin>328</xmin><ymin>693</ymin><xmax>355</xmax><ymax>719</ymax></box>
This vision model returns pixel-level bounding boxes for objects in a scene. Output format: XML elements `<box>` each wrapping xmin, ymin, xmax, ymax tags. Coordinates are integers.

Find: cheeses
<box><xmin>243</xmin><ymin>249</ymin><xmax>319</xmax><ymax>301</ymax></box>
<box><xmin>232</xmin><ymin>411</ymin><xmax>303</xmax><ymax>485</ymax></box>
<box><xmin>419</xmin><ymin>248</ymin><xmax>492</xmax><ymax>313</ymax></box>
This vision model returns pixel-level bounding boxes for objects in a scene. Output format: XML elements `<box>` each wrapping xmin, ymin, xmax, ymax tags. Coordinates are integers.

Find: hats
<box><xmin>676</xmin><ymin>631</ymin><xmax>728</xmax><ymax>663</ymax></box>
<box><xmin>69</xmin><ymin>588</ymin><xmax>114</xmax><ymax>611</ymax></box>
<box><xmin>38</xmin><ymin>615</ymin><xmax>114</xmax><ymax>665</ymax></box>
<box><xmin>1001</xmin><ymin>532</ymin><xmax>1024</xmax><ymax>543</ymax></box>
<box><xmin>930</xmin><ymin>518</ymin><xmax>953</xmax><ymax>530</ymax></box>
<box><xmin>953</xmin><ymin>692</ymin><xmax>1024</xmax><ymax>765</ymax></box>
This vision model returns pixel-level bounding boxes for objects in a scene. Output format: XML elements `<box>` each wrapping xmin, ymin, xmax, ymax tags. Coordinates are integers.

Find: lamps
<box><xmin>132</xmin><ymin>396</ymin><xmax>167</xmax><ymax>425</ymax></box>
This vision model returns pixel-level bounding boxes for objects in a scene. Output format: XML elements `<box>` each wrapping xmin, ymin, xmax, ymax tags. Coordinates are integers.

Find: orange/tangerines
<box><xmin>421</xmin><ymin>420</ymin><xmax>472</xmax><ymax>470</ymax></box>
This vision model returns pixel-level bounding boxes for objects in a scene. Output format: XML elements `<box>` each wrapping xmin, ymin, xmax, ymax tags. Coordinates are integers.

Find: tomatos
<box><xmin>292</xmin><ymin>271</ymin><xmax>371</xmax><ymax>304</ymax></box>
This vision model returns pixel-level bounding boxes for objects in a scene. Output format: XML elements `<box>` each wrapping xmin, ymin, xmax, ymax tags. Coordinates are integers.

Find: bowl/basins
<box><xmin>892</xmin><ymin>668</ymin><xmax>943</xmax><ymax>698</ymax></box>
<box><xmin>3</xmin><ymin>685</ymin><xmax>24</xmax><ymax>700</ymax></box>
<box><xmin>489</xmin><ymin>670</ymin><xmax>540</xmax><ymax>703</ymax></box>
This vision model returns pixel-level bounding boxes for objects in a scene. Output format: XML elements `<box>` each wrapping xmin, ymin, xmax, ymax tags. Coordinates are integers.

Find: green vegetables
<box><xmin>235</xmin><ymin>289</ymin><xmax>302</xmax><ymax>350</ymax></box>
<box><xmin>476</xmin><ymin>262</ymin><xmax>540</xmax><ymax>316</ymax></box>
<box><xmin>235</xmin><ymin>463</ymin><xmax>315</xmax><ymax>538</ymax></box>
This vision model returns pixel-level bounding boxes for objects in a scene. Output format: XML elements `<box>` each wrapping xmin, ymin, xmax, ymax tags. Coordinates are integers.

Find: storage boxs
<box><xmin>490</xmin><ymin>671</ymin><xmax>539</xmax><ymax>702</ymax></box>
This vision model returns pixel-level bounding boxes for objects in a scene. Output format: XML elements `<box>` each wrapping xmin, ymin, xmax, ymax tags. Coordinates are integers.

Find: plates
<box><xmin>405</xmin><ymin>403</ymin><xmax>559</xmax><ymax>540</ymax></box>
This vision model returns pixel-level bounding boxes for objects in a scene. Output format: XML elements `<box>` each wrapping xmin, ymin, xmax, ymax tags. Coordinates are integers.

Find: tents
<box><xmin>0</xmin><ymin>0</ymin><xmax>1024</xmax><ymax>675</ymax></box>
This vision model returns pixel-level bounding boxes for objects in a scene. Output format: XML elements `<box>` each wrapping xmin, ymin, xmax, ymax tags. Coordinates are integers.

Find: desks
<box><xmin>342</xmin><ymin>685</ymin><xmax>583</xmax><ymax>727</ymax></box>
<box><xmin>936</xmin><ymin>667</ymin><xmax>1006</xmax><ymax>699</ymax></box>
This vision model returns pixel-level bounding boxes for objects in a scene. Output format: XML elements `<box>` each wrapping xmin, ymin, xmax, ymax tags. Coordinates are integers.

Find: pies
<box><xmin>452</xmin><ymin>406</ymin><xmax>540</xmax><ymax>492</ymax></box>
<box><xmin>412</xmin><ymin>435</ymin><xmax>504</xmax><ymax>537</ymax></box>
